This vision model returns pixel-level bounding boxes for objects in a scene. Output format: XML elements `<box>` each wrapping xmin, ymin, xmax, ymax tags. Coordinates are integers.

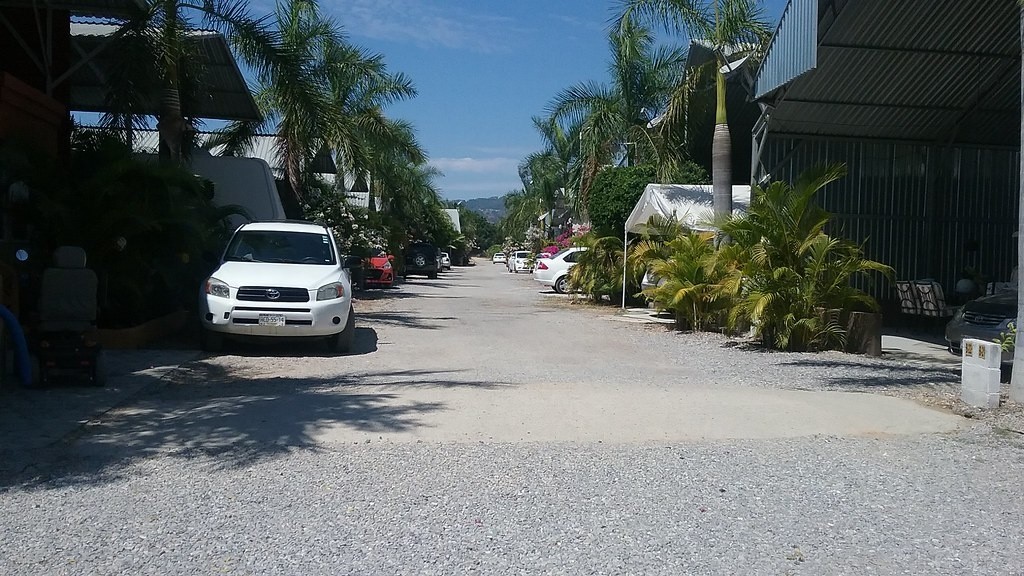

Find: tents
<box><xmin>622</xmin><ymin>183</ymin><xmax>751</xmax><ymax>311</ymax></box>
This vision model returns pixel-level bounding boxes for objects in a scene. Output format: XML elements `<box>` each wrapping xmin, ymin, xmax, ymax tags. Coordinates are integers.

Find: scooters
<box><xmin>15</xmin><ymin>249</ymin><xmax>106</xmax><ymax>389</ymax></box>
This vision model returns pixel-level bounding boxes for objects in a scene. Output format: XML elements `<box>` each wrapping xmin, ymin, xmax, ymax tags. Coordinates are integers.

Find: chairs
<box><xmin>896</xmin><ymin>280</ymin><xmax>961</xmax><ymax>338</ymax></box>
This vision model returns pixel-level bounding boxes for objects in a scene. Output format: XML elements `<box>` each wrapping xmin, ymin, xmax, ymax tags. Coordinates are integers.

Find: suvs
<box><xmin>197</xmin><ymin>219</ymin><xmax>356</xmax><ymax>354</ymax></box>
<box><xmin>397</xmin><ymin>242</ymin><xmax>438</xmax><ymax>279</ymax></box>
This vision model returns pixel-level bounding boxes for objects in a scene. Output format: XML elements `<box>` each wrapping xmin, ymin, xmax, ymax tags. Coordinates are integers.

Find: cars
<box><xmin>531</xmin><ymin>246</ymin><xmax>591</xmax><ymax>294</ymax></box>
<box><xmin>492</xmin><ymin>253</ymin><xmax>509</xmax><ymax>264</ymax></box>
<box><xmin>436</xmin><ymin>247</ymin><xmax>451</xmax><ymax>273</ymax></box>
<box><xmin>508</xmin><ymin>250</ymin><xmax>534</xmax><ymax>273</ymax></box>
<box><xmin>943</xmin><ymin>284</ymin><xmax>1018</xmax><ymax>365</ymax></box>
<box><xmin>358</xmin><ymin>250</ymin><xmax>395</xmax><ymax>288</ymax></box>
